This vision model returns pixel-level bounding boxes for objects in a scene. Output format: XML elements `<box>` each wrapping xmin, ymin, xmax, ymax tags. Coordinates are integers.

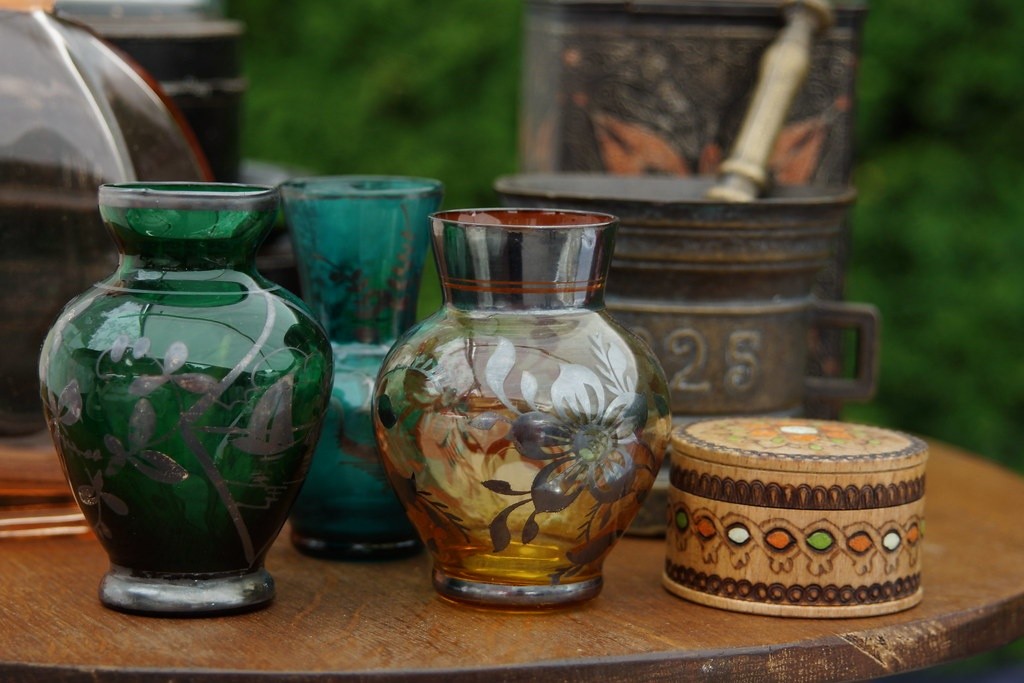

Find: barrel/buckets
<box><xmin>488</xmin><ymin>171</ymin><xmax>880</xmax><ymax>542</ymax></box>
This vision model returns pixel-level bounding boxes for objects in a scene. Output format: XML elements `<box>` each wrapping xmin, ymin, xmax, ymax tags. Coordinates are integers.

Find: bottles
<box><xmin>370</xmin><ymin>208</ymin><xmax>673</xmax><ymax>610</ymax></box>
<box><xmin>35</xmin><ymin>180</ymin><xmax>337</xmax><ymax>618</ymax></box>
<box><xmin>272</xmin><ymin>175</ymin><xmax>451</xmax><ymax>556</ymax></box>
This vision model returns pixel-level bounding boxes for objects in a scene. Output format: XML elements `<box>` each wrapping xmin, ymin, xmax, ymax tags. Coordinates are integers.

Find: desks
<box><xmin>0</xmin><ymin>426</ymin><xmax>1024</xmax><ymax>683</ymax></box>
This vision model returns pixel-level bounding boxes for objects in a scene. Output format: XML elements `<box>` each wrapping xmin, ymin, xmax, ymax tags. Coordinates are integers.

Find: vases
<box><xmin>372</xmin><ymin>206</ymin><xmax>674</xmax><ymax>610</ymax></box>
<box><xmin>37</xmin><ymin>181</ymin><xmax>334</xmax><ymax>615</ymax></box>
<box><xmin>285</xmin><ymin>172</ymin><xmax>447</xmax><ymax>561</ymax></box>
